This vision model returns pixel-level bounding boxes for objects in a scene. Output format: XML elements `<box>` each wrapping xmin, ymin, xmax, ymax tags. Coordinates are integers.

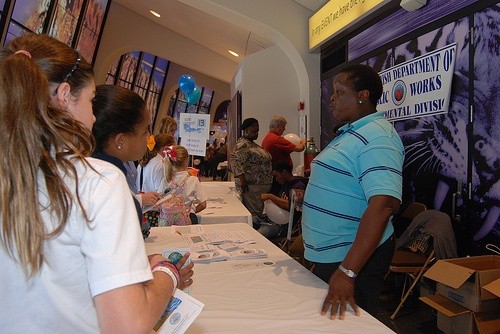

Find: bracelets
<box><xmin>151</xmin><ymin>261</ymin><xmax>181</xmax><ymax>287</ymax></box>
<box><xmin>151</xmin><ymin>266</ymin><xmax>178</xmax><ymax>291</ymax></box>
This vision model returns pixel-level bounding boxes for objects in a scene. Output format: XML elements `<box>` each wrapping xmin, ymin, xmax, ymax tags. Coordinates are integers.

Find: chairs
<box><xmin>390</xmin><ymin>210</ymin><xmax>458</xmax><ymax>319</ymax></box>
<box><xmin>279</xmin><ymin>216</ymin><xmax>302</xmax><ymax>249</ymax></box>
<box><xmin>401</xmin><ymin>202</ymin><xmax>427</xmax><ymax>220</ymax></box>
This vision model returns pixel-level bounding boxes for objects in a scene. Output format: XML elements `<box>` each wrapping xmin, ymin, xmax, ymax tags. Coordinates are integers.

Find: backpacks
<box><xmin>157</xmin><ymin>173</ymin><xmax>193</xmax><ymax>226</ymax></box>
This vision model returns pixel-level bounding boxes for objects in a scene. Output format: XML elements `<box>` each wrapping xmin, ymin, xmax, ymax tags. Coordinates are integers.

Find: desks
<box><xmin>195</xmin><ymin>184</ymin><xmax>253</xmax><ymax>227</ymax></box>
<box><xmin>145</xmin><ymin>223</ymin><xmax>397</xmax><ymax>334</ymax></box>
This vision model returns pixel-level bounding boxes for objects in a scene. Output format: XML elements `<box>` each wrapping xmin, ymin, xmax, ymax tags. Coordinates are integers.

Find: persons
<box><xmin>190</xmin><ymin>140</ymin><xmax>229</xmax><ymax>182</ymax></box>
<box><xmin>301</xmin><ymin>64</ymin><xmax>405</xmax><ymax>321</ymax></box>
<box><xmin>227</xmin><ymin>118</ymin><xmax>273</xmax><ymax>231</ymax></box>
<box><xmin>252</xmin><ymin>161</ymin><xmax>307</xmax><ymax>240</ymax></box>
<box><xmin>260</xmin><ymin>114</ymin><xmax>308</xmax><ymax>173</ymax></box>
<box><xmin>91</xmin><ymin>83</ymin><xmax>194</xmax><ymax>290</ymax></box>
<box><xmin>0</xmin><ymin>35</ymin><xmax>180</xmax><ymax>334</ymax></box>
<box><xmin>124</xmin><ymin>115</ymin><xmax>207</xmax><ymax>237</ymax></box>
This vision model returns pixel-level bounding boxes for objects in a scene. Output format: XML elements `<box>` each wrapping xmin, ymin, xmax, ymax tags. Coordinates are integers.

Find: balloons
<box><xmin>209</xmin><ymin>124</ymin><xmax>228</xmax><ymax>148</ymax></box>
<box><xmin>184</xmin><ymin>85</ymin><xmax>201</xmax><ymax>105</ymax></box>
<box><xmin>264</xmin><ymin>197</ymin><xmax>289</xmax><ymax>225</ymax></box>
<box><xmin>284</xmin><ymin>133</ymin><xmax>301</xmax><ymax>146</ymax></box>
<box><xmin>178</xmin><ymin>74</ymin><xmax>195</xmax><ymax>95</ymax></box>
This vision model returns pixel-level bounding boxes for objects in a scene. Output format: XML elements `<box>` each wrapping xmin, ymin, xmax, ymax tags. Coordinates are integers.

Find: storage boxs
<box><xmin>423</xmin><ymin>255</ymin><xmax>500</xmax><ymax>313</ymax></box>
<box><xmin>418</xmin><ymin>294</ymin><xmax>500</xmax><ymax>334</ymax></box>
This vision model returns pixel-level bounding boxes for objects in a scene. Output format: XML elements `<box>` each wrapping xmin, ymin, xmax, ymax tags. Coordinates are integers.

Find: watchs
<box><xmin>336</xmin><ymin>263</ymin><xmax>358</xmax><ymax>280</ymax></box>
